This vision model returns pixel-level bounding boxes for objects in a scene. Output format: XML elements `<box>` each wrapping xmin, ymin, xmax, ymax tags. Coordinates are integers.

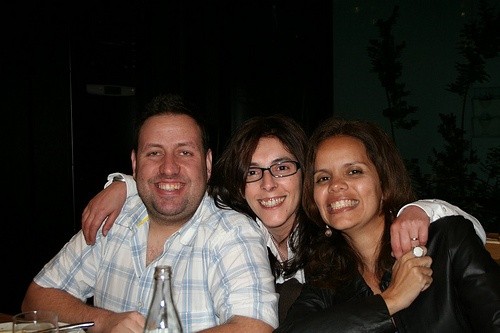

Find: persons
<box><xmin>82</xmin><ymin>113</ymin><xmax>486</xmax><ymax>333</ymax></box>
<box><xmin>22</xmin><ymin>94</ymin><xmax>280</xmax><ymax>333</ymax></box>
<box><xmin>283</xmin><ymin>118</ymin><xmax>500</xmax><ymax>333</ymax></box>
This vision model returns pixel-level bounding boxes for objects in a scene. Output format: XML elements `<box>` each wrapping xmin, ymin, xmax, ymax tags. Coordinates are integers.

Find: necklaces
<box><xmin>269</xmin><ymin>230</ymin><xmax>288</xmax><ymax>247</ymax></box>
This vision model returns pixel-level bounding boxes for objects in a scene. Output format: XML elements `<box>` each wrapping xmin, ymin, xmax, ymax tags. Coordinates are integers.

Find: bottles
<box><xmin>144</xmin><ymin>265</ymin><xmax>183</xmax><ymax>333</ymax></box>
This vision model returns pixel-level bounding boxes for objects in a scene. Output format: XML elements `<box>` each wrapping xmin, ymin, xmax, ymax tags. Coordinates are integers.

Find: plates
<box><xmin>0</xmin><ymin>321</ymin><xmax>86</xmax><ymax>333</ymax></box>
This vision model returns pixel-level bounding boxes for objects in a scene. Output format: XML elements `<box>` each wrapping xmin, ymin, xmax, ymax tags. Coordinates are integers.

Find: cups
<box><xmin>12</xmin><ymin>309</ymin><xmax>59</xmax><ymax>333</ymax></box>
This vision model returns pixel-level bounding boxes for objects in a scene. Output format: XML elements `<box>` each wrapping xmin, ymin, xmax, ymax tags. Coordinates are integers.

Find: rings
<box><xmin>413</xmin><ymin>247</ymin><xmax>423</xmax><ymax>257</ymax></box>
<box><xmin>410</xmin><ymin>238</ymin><xmax>419</xmax><ymax>240</ymax></box>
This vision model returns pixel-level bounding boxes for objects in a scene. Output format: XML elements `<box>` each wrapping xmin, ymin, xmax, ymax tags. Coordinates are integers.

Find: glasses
<box><xmin>246</xmin><ymin>161</ymin><xmax>301</xmax><ymax>183</ymax></box>
